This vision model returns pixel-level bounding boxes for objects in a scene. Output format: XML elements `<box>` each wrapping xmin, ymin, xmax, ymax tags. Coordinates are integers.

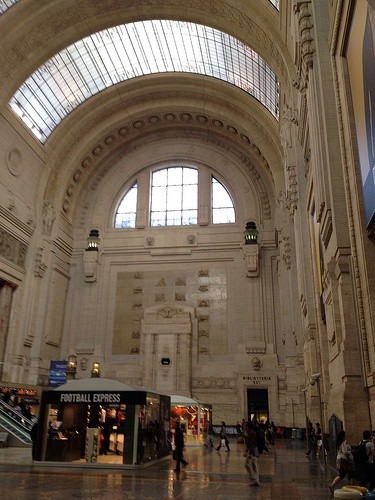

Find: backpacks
<box><xmin>351</xmin><ymin>440</ymin><xmax>371</xmax><ymax>464</ymax></box>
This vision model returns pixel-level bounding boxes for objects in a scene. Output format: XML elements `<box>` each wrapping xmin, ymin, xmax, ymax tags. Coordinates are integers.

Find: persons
<box><xmin>204</xmin><ymin>421</ymin><xmax>217</xmax><ymax>448</ymax></box>
<box><xmin>329</xmin><ymin>430</ymin><xmax>375</xmax><ymax>496</ymax></box>
<box><xmin>0</xmin><ymin>391</ymin><xmax>36</xmax><ymax>429</ymax></box>
<box><xmin>172</xmin><ymin>421</ymin><xmax>188</xmax><ymax>472</ymax></box>
<box><xmin>235</xmin><ymin>418</ymin><xmax>283</xmax><ymax>458</ymax></box>
<box><xmin>305</xmin><ymin>422</ymin><xmax>328</xmax><ymax>457</ymax></box>
<box><xmin>215</xmin><ymin>421</ymin><xmax>230</xmax><ymax>453</ymax></box>
<box><xmin>245</xmin><ymin>420</ymin><xmax>262</xmax><ymax>487</ymax></box>
<box><xmin>31</xmin><ymin>423</ymin><xmax>38</xmax><ymax>460</ymax></box>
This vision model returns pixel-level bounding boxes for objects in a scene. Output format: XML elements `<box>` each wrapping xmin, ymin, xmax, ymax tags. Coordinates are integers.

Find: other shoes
<box><xmin>249</xmin><ymin>482</ymin><xmax>260</xmax><ymax>487</ymax></box>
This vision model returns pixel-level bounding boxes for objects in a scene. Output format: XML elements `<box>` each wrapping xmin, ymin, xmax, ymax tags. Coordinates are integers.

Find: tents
<box><xmin>171</xmin><ymin>394</ymin><xmax>213</xmax><ymax>446</ymax></box>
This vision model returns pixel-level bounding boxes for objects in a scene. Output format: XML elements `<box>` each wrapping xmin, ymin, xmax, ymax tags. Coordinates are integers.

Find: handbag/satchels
<box><xmin>172</xmin><ymin>450</ymin><xmax>184</xmax><ymax>460</ymax></box>
<box><xmin>339</xmin><ymin>458</ymin><xmax>353</xmax><ymax>472</ymax></box>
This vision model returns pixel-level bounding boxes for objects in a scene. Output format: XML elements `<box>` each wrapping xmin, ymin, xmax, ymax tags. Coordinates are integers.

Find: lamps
<box><xmin>243</xmin><ymin>221</ymin><xmax>258</xmax><ymax>245</ymax></box>
<box><xmin>161</xmin><ymin>358</ymin><xmax>170</xmax><ymax>365</ymax></box>
<box><xmin>92</xmin><ymin>363</ymin><xmax>99</xmax><ymax>374</ymax></box>
<box><xmin>67</xmin><ymin>355</ymin><xmax>77</xmax><ymax>369</ymax></box>
<box><xmin>86</xmin><ymin>230</ymin><xmax>100</xmax><ymax>251</ymax></box>
